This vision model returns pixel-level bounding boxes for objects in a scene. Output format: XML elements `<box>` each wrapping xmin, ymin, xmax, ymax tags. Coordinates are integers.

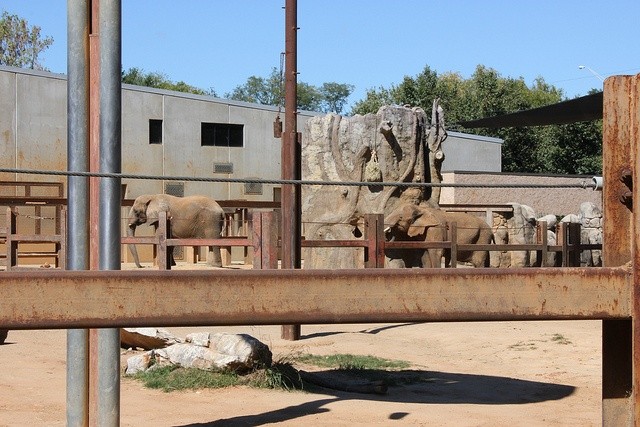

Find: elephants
<box><xmin>384</xmin><ymin>203</ymin><xmax>498</xmax><ymax>268</ymax></box>
<box><xmin>123</xmin><ymin>191</ymin><xmax>231</xmax><ymax>268</ymax></box>
